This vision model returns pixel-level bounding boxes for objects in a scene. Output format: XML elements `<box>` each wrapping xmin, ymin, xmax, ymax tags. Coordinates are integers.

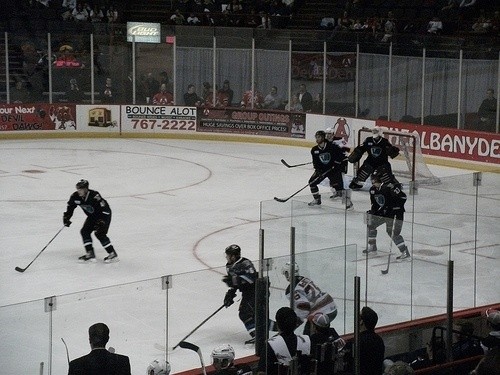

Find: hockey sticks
<box><xmin>380</xmin><ymin>214</ymin><xmax>396</xmax><ymax>274</ymax></box>
<box><xmin>169</xmin><ymin>304</ymin><xmax>224</xmax><ymax>351</ymax></box>
<box><xmin>15</xmin><ymin>221</ymin><xmax>67</xmax><ymax>274</ymax></box>
<box><xmin>280</xmin><ymin>158</ymin><xmax>313</xmax><ymax>169</ymax></box>
<box><xmin>60</xmin><ymin>336</ymin><xmax>70</xmax><ymax>362</ymax></box>
<box><xmin>178</xmin><ymin>341</ymin><xmax>207</xmax><ymax>375</ymax></box>
<box><xmin>272</xmin><ymin>158</ymin><xmax>349</xmax><ymax>203</ymax></box>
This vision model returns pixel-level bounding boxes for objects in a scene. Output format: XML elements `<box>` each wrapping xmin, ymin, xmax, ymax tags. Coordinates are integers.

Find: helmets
<box><xmin>325</xmin><ymin>127</ymin><xmax>335</xmax><ymax>133</ymax></box>
<box><xmin>76</xmin><ymin>179</ymin><xmax>89</xmax><ymax>189</ymax></box>
<box><xmin>282</xmin><ymin>261</ymin><xmax>300</xmax><ymax>277</ymax></box>
<box><xmin>373</xmin><ymin>127</ymin><xmax>383</xmax><ymax>138</ymax></box>
<box><xmin>212</xmin><ymin>344</ymin><xmax>235</xmax><ymax>362</ymax></box>
<box><xmin>315</xmin><ymin>130</ymin><xmax>326</xmax><ymax>137</ymax></box>
<box><xmin>147</xmin><ymin>360</ymin><xmax>171</xmax><ymax>375</ymax></box>
<box><xmin>372</xmin><ymin>169</ymin><xmax>383</xmax><ymax>181</ymax></box>
<box><xmin>225</xmin><ymin>245</ymin><xmax>241</xmax><ymax>255</ymax></box>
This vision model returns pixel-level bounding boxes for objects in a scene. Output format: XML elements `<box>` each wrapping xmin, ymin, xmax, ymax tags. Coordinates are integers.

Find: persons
<box><xmin>124</xmin><ymin>68</ymin><xmax>324</xmax><ymax>114</ymax></box>
<box><xmin>308</xmin><ymin>131</ymin><xmax>353</xmax><ymax>210</ymax></box>
<box><xmin>340</xmin><ymin>307</ymin><xmax>500</xmax><ymax>375</ymax></box>
<box><xmin>475</xmin><ymin>88</ymin><xmax>496</xmax><ymax>133</ymax></box>
<box><xmin>343</xmin><ymin>126</ymin><xmax>403</xmax><ymax>191</ymax></box>
<box><xmin>62</xmin><ymin>179</ymin><xmax>118</xmax><ymax>263</ymax></box>
<box><xmin>324</xmin><ymin>128</ymin><xmax>346</xmax><ymax>198</ymax></box>
<box><xmin>221</xmin><ymin>244</ymin><xmax>278</xmax><ymax>346</ymax></box>
<box><xmin>362</xmin><ymin>171</ymin><xmax>411</xmax><ymax>262</ymax></box>
<box><xmin>7</xmin><ymin>0</ymin><xmax>120</xmax><ymax>103</ymax></box>
<box><xmin>309</xmin><ymin>313</ymin><xmax>346</xmax><ymax>355</ymax></box>
<box><xmin>67</xmin><ymin>323</ymin><xmax>131</xmax><ymax>375</ymax></box>
<box><xmin>280</xmin><ymin>261</ymin><xmax>338</xmax><ymax>335</ymax></box>
<box><xmin>317</xmin><ymin>0</ymin><xmax>500</xmax><ymax>49</ymax></box>
<box><xmin>210</xmin><ymin>344</ymin><xmax>253</xmax><ymax>375</ymax></box>
<box><xmin>257</xmin><ymin>306</ymin><xmax>315</xmax><ymax>375</ymax></box>
<box><xmin>166</xmin><ymin>0</ymin><xmax>303</xmax><ymax>41</ymax></box>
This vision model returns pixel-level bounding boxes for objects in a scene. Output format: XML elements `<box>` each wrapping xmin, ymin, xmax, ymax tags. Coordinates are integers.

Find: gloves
<box><xmin>314</xmin><ymin>171</ymin><xmax>320</xmax><ymax>181</ymax></box>
<box><xmin>378</xmin><ymin>205</ymin><xmax>393</xmax><ymax>217</ymax></box>
<box><xmin>332</xmin><ymin>162</ymin><xmax>340</xmax><ymax>171</ymax></box>
<box><xmin>93</xmin><ymin>221</ymin><xmax>106</xmax><ymax>231</ymax></box>
<box><xmin>224</xmin><ymin>289</ymin><xmax>236</xmax><ymax>308</ymax></box>
<box><xmin>222</xmin><ymin>275</ymin><xmax>235</xmax><ymax>287</ymax></box>
<box><xmin>63</xmin><ymin>213</ymin><xmax>72</xmax><ymax>228</ymax></box>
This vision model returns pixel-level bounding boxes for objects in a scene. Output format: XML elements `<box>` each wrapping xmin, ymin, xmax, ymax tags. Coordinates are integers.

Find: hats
<box><xmin>308</xmin><ymin>313</ymin><xmax>329</xmax><ymax>328</ymax></box>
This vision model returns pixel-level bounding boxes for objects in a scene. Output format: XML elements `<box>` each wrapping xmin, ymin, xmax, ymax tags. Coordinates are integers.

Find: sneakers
<box><xmin>362</xmin><ymin>243</ymin><xmax>377</xmax><ymax>256</ymax></box>
<box><xmin>104</xmin><ymin>252</ymin><xmax>119</xmax><ymax>263</ymax></box>
<box><xmin>347</xmin><ymin>197</ymin><xmax>354</xmax><ymax>211</ymax></box>
<box><xmin>308</xmin><ymin>196</ymin><xmax>322</xmax><ymax>207</ymax></box>
<box><xmin>396</xmin><ymin>246</ymin><xmax>411</xmax><ymax>263</ymax></box>
<box><xmin>330</xmin><ymin>193</ymin><xmax>340</xmax><ymax>199</ymax></box>
<box><xmin>78</xmin><ymin>248</ymin><xmax>96</xmax><ymax>263</ymax></box>
